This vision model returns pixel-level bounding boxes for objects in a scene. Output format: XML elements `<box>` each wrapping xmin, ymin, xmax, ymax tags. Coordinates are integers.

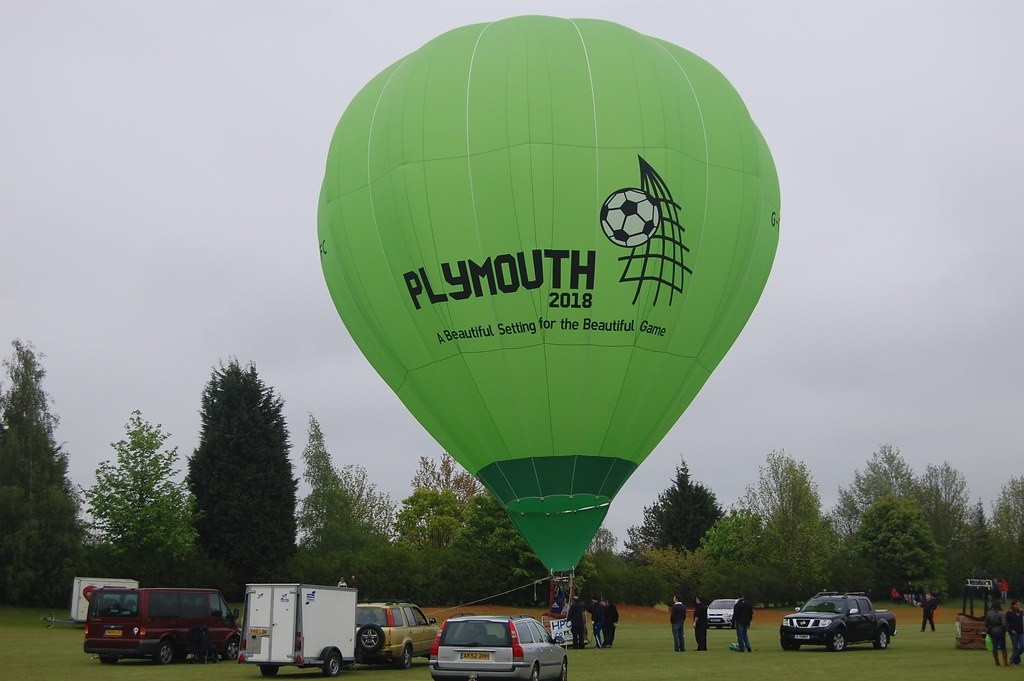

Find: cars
<box><xmin>706</xmin><ymin>599</ymin><xmax>749</xmax><ymax>629</ymax></box>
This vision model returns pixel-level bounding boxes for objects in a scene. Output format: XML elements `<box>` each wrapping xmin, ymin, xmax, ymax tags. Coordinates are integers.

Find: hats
<box><xmin>592</xmin><ymin>595</ymin><xmax>601</xmax><ymax>602</ymax></box>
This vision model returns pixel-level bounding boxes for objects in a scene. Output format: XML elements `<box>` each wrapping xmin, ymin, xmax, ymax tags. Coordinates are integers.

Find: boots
<box><xmin>993</xmin><ymin>652</ymin><xmax>1000</xmax><ymax>665</ymax></box>
<box><xmin>1002</xmin><ymin>652</ymin><xmax>1008</xmax><ymax>665</ymax></box>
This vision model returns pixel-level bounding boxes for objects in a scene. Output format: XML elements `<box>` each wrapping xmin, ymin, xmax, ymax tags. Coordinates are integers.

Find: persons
<box><xmin>693</xmin><ymin>596</ymin><xmax>708</xmax><ymax>651</ymax></box>
<box><xmin>984</xmin><ymin>579</ymin><xmax>1024</xmax><ymax>665</ymax></box>
<box><xmin>561</xmin><ymin>596</ymin><xmax>620</xmax><ymax>649</ymax></box>
<box><xmin>670</xmin><ymin>596</ymin><xmax>686</xmax><ymax>652</ymax></box>
<box><xmin>338</xmin><ymin>576</ymin><xmax>358</xmax><ymax>588</ymax></box>
<box><xmin>731</xmin><ymin>592</ymin><xmax>753</xmax><ymax>652</ymax></box>
<box><xmin>891</xmin><ymin>581</ymin><xmax>937</xmax><ymax>632</ymax></box>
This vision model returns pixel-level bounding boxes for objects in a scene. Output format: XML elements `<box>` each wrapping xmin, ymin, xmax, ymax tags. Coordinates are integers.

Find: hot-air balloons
<box><xmin>316</xmin><ymin>13</ymin><xmax>782</xmax><ymax>647</ymax></box>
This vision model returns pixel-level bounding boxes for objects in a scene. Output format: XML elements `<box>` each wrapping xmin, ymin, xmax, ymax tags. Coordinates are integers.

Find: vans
<box><xmin>84</xmin><ymin>585</ymin><xmax>242</xmax><ymax>666</ymax></box>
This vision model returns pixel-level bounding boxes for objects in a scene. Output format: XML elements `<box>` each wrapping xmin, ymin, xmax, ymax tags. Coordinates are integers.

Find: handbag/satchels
<box><xmin>985</xmin><ymin>633</ymin><xmax>994</xmax><ymax>652</ymax></box>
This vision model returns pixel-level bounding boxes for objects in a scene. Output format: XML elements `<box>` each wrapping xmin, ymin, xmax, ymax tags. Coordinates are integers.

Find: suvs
<box><xmin>429</xmin><ymin>612</ymin><xmax>568</xmax><ymax>681</ymax></box>
<box><xmin>354</xmin><ymin>599</ymin><xmax>440</xmax><ymax>669</ymax></box>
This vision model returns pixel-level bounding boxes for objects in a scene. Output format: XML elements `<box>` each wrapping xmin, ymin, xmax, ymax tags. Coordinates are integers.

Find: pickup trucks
<box><xmin>780</xmin><ymin>589</ymin><xmax>898</xmax><ymax>653</ymax></box>
